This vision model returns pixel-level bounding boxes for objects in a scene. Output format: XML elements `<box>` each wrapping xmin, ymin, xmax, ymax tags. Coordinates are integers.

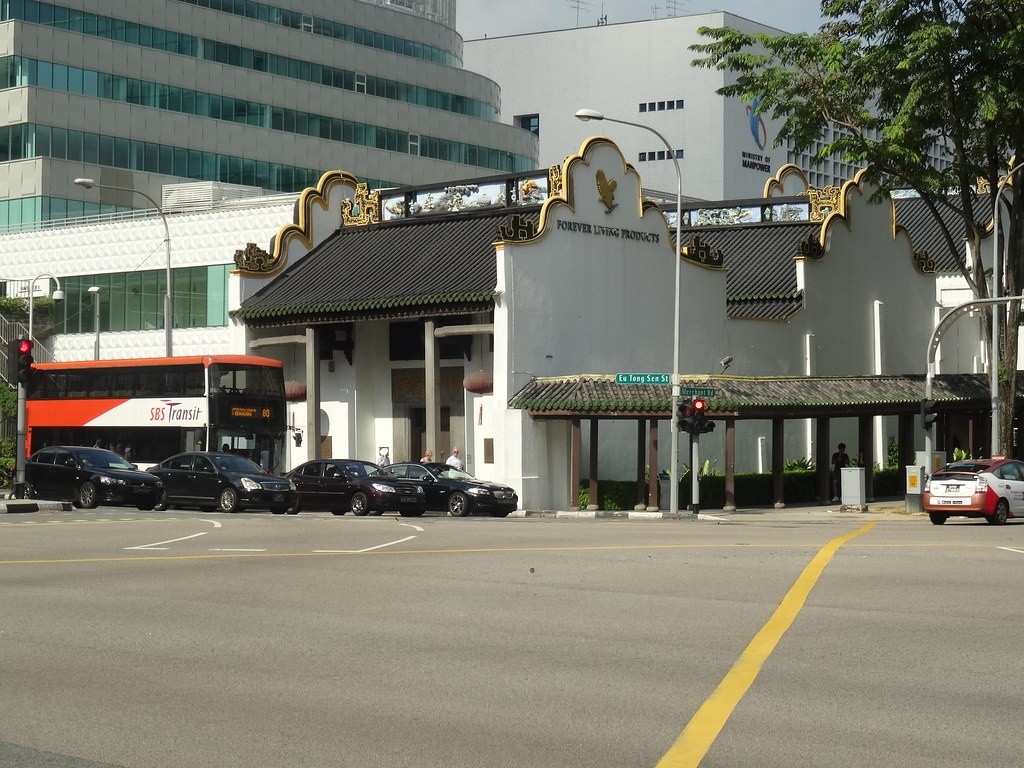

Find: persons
<box><xmin>80</xmin><ymin>438</ymin><xmax>136</xmax><ymax>460</ymax></box>
<box><xmin>832</xmin><ymin>443</ymin><xmax>850</xmax><ymax>501</ymax></box>
<box><xmin>9</xmin><ymin>464</ymin><xmax>16</xmax><ymax>500</ymax></box>
<box><xmin>446</xmin><ymin>447</ymin><xmax>464</xmax><ymax>479</ymax></box>
<box><xmin>378</xmin><ymin>452</ymin><xmax>392</xmax><ymax>474</ymax></box>
<box><xmin>420</xmin><ymin>449</ymin><xmax>432</xmax><ymax>463</ymax></box>
<box><xmin>212</xmin><ymin>376</ymin><xmax>226</xmax><ymax>392</ymax></box>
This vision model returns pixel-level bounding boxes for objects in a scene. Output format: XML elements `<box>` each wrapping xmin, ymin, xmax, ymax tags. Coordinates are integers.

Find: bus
<box><xmin>24</xmin><ymin>355</ymin><xmax>304</xmax><ymax>473</ymax></box>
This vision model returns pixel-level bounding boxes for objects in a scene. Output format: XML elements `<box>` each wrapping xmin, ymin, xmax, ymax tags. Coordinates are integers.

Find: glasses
<box><xmin>454</xmin><ymin>452</ymin><xmax>459</xmax><ymax>453</ymax></box>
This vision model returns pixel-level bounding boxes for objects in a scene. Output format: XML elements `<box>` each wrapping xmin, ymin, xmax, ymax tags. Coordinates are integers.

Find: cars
<box><xmin>922</xmin><ymin>458</ymin><xmax>1024</xmax><ymax>525</ymax></box>
<box><xmin>276</xmin><ymin>459</ymin><xmax>425</xmax><ymax>517</ymax></box>
<box><xmin>144</xmin><ymin>450</ymin><xmax>297</xmax><ymax>514</ymax></box>
<box><xmin>11</xmin><ymin>445</ymin><xmax>164</xmax><ymax>508</ymax></box>
<box><xmin>368</xmin><ymin>461</ymin><xmax>518</xmax><ymax>517</ymax></box>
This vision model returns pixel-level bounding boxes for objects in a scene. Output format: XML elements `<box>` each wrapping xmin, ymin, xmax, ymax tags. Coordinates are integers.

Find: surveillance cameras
<box><xmin>51</xmin><ymin>291</ymin><xmax>65</xmax><ymax>303</ymax></box>
<box><xmin>720</xmin><ymin>356</ymin><xmax>734</xmax><ymax>366</ymax></box>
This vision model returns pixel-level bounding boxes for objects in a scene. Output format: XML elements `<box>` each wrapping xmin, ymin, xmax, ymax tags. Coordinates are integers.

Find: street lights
<box><xmin>72</xmin><ymin>178</ymin><xmax>174</xmax><ymax>355</ymax></box>
<box><xmin>88</xmin><ymin>287</ymin><xmax>100</xmax><ymax>360</ymax></box>
<box><xmin>573</xmin><ymin>108</ymin><xmax>682</xmax><ymax>513</ymax></box>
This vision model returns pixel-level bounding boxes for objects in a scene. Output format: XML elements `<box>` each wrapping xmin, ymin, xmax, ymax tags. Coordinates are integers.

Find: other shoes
<box><xmin>831</xmin><ymin>496</ymin><xmax>839</xmax><ymax>501</ymax></box>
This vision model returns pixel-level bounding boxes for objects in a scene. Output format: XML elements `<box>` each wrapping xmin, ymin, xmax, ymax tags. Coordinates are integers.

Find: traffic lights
<box><xmin>679</xmin><ymin>402</ymin><xmax>693</xmax><ymax>432</ymax></box>
<box><xmin>920</xmin><ymin>397</ymin><xmax>937</xmax><ymax>430</ymax></box>
<box><xmin>18</xmin><ymin>339</ymin><xmax>33</xmax><ymax>382</ymax></box>
<box><xmin>693</xmin><ymin>399</ymin><xmax>716</xmax><ymax>433</ymax></box>
